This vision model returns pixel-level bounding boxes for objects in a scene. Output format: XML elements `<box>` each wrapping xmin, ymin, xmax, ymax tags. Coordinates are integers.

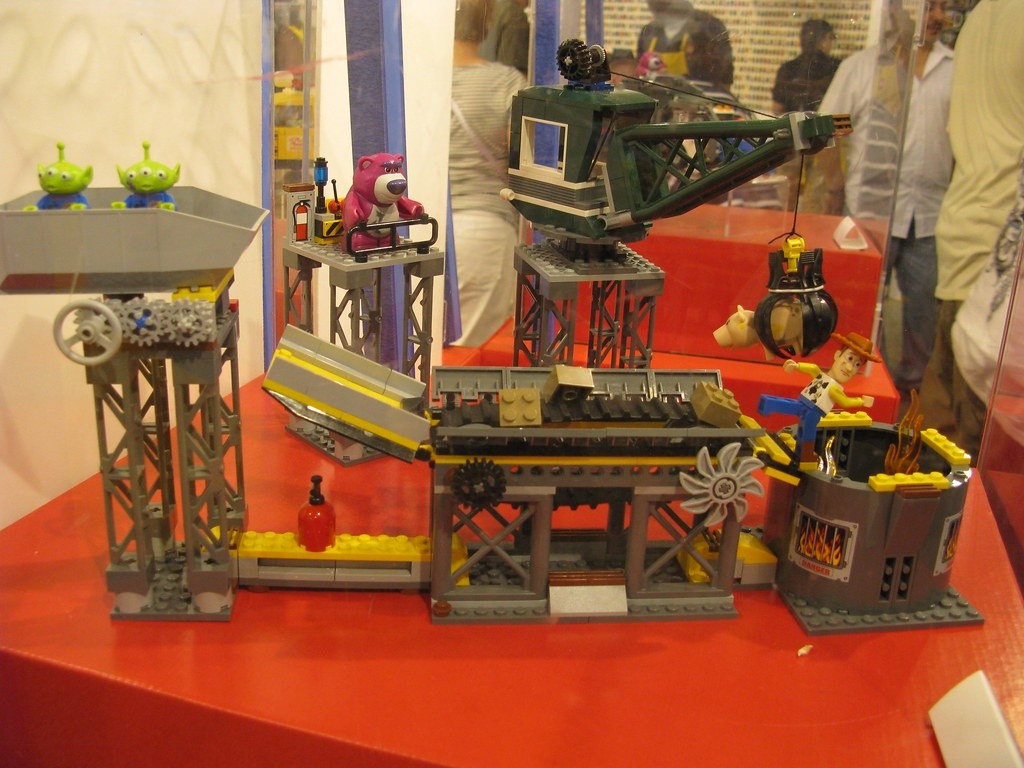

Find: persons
<box><xmin>478</xmin><ymin>0</ymin><xmax>529</xmax><ymax>80</ymax></box>
<box><xmin>911</xmin><ymin>0</ymin><xmax>1024</xmax><ymax>473</ymax></box>
<box><xmin>448</xmin><ymin>0</ymin><xmax>530</xmax><ymax>348</ymax></box>
<box><xmin>637</xmin><ymin>0</ymin><xmax>735</xmax><ymax>121</ymax></box>
<box><xmin>757</xmin><ymin>330</ymin><xmax>884</xmax><ymax>469</ymax></box>
<box><xmin>771</xmin><ymin>18</ymin><xmax>846</xmax><ymax>217</ymax></box>
<box><xmin>799</xmin><ymin>1</ymin><xmax>959</xmax><ymax>401</ymax></box>
